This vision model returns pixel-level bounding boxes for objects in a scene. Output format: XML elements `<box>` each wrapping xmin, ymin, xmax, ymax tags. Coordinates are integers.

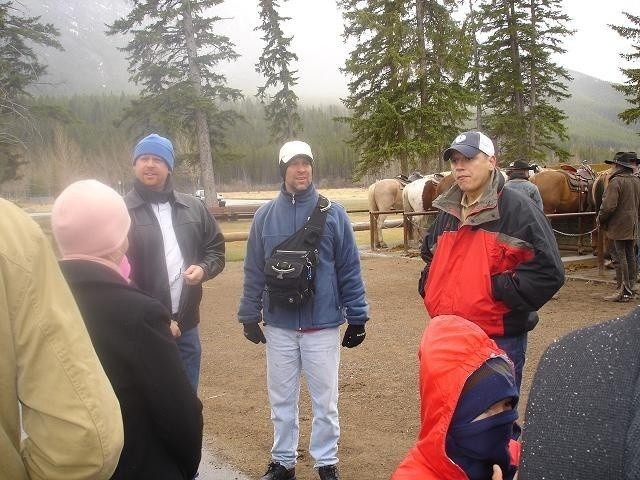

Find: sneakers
<box><xmin>315</xmin><ymin>465</ymin><xmax>342</xmax><ymax>479</ymax></box>
<box><xmin>259</xmin><ymin>462</ymin><xmax>298</xmax><ymax>480</ymax></box>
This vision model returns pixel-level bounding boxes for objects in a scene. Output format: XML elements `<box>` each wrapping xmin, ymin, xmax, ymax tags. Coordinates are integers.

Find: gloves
<box><xmin>242</xmin><ymin>322</ymin><xmax>269</xmax><ymax>344</ymax></box>
<box><xmin>342</xmin><ymin>323</ymin><xmax>367</xmax><ymax>348</ymax></box>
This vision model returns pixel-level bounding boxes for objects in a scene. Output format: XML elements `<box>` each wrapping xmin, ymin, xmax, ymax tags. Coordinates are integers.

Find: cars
<box><xmin>192</xmin><ymin>189</ymin><xmax>227</xmax><ymax>207</ymax></box>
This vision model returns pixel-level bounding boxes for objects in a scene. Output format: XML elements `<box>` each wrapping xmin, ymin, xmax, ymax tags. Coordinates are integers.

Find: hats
<box><xmin>277</xmin><ymin>141</ymin><xmax>315</xmax><ymax>183</ymax></box>
<box><xmin>131</xmin><ymin>133</ymin><xmax>176</xmax><ymax>177</ymax></box>
<box><xmin>603</xmin><ymin>150</ymin><xmax>640</xmax><ymax>170</ymax></box>
<box><xmin>450</xmin><ymin>360</ymin><xmax>521</xmax><ymax>435</ymax></box>
<box><xmin>503</xmin><ymin>159</ymin><xmax>534</xmax><ymax>172</ymax></box>
<box><xmin>442</xmin><ymin>130</ymin><xmax>495</xmax><ymax>162</ymax></box>
<box><xmin>49</xmin><ymin>178</ymin><xmax>133</xmax><ymax>260</ymax></box>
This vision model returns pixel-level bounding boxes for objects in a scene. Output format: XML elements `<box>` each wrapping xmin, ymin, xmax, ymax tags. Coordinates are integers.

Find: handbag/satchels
<box><xmin>261</xmin><ymin>195</ymin><xmax>332</xmax><ymax>316</ymax></box>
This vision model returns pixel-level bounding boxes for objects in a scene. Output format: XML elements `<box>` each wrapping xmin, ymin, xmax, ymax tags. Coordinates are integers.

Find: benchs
<box><xmin>230</xmin><ymin>204</ymin><xmax>260</xmax><ymax>219</ymax></box>
<box><xmin>207</xmin><ymin>208</ymin><xmax>231</xmax><ymax>221</ymax></box>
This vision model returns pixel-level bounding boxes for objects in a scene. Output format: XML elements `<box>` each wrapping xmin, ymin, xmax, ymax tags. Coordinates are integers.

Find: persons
<box><xmin>629</xmin><ymin>152</ymin><xmax>640</xmax><ymax>283</ymax></box>
<box><xmin>0</xmin><ymin>197</ymin><xmax>124</xmax><ymax>480</ymax></box>
<box><xmin>237</xmin><ymin>140</ymin><xmax>370</xmax><ymax>480</ymax></box>
<box><xmin>596</xmin><ymin>151</ymin><xmax>640</xmax><ymax>303</ymax></box>
<box><xmin>51</xmin><ymin>178</ymin><xmax>203</xmax><ymax>480</ymax></box>
<box><xmin>418</xmin><ymin>131</ymin><xmax>565</xmax><ymax>393</ymax></box>
<box><xmin>390</xmin><ymin>315</ymin><xmax>522</xmax><ymax>480</ymax></box>
<box><xmin>506</xmin><ymin>162</ymin><xmax>514</xmax><ymax>183</ymax></box>
<box><xmin>124</xmin><ymin>133</ymin><xmax>225</xmax><ymax>396</ymax></box>
<box><xmin>518</xmin><ymin>306</ymin><xmax>640</xmax><ymax>480</ymax></box>
<box><xmin>503</xmin><ymin>159</ymin><xmax>543</xmax><ymax>211</ymax></box>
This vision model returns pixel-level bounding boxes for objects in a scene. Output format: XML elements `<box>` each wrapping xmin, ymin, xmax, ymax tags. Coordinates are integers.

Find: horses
<box><xmin>368</xmin><ymin>170</ymin><xmax>458</xmax><ymax>259</ymax></box>
<box><xmin>500</xmin><ymin>165</ymin><xmax>616</xmax><ymax>268</ymax></box>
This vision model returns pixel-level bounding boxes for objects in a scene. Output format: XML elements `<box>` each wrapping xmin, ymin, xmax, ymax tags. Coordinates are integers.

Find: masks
<box><xmin>449</xmin><ymin>409</ymin><xmax>521</xmax><ymax>464</ymax></box>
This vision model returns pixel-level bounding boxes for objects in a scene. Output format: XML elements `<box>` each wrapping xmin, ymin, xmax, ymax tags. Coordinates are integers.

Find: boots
<box><xmin>602</xmin><ymin>282</ymin><xmax>631</xmax><ymax>303</ymax></box>
